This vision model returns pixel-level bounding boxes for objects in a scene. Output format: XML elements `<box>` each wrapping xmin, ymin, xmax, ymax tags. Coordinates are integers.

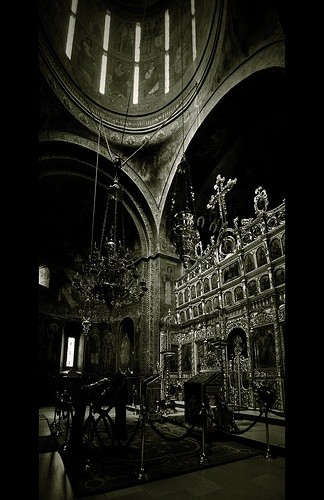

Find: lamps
<box><xmin>71</xmin><ymin>156</ymin><xmax>147</xmax><ymax>323</ymax></box>
